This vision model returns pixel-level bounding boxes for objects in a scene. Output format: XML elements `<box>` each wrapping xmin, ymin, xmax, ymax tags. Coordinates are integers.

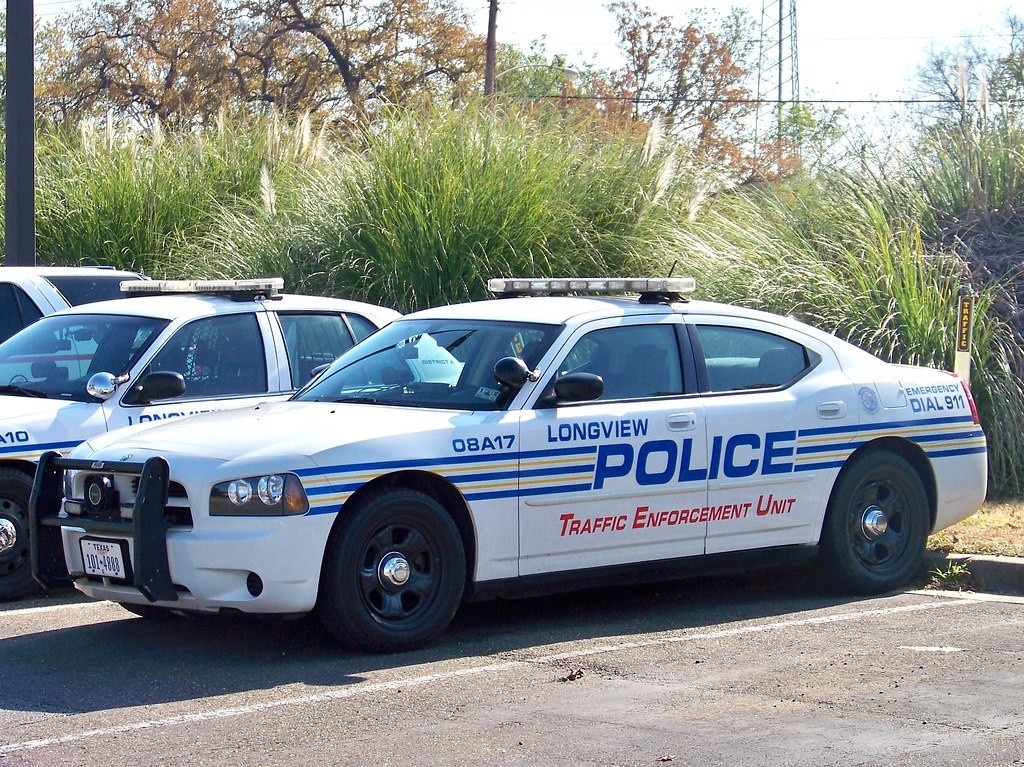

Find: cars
<box><xmin>1</xmin><ymin>262</ymin><xmax>173</xmax><ymax>378</ymax></box>
<box><xmin>27</xmin><ymin>275</ymin><xmax>988</xmax><ymax>657</ymax></box>
<box><xmin>0</xmin><ymin>279</ymin><xmax>464</xmax><ymax>600</ymax></box>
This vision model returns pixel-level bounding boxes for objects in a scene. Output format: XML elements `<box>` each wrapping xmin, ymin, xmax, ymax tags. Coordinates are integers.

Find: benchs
<box><xmin>616</xmin><ymin>345</ymin><xmax>800</xmax><ymax>391</ymax></box>
